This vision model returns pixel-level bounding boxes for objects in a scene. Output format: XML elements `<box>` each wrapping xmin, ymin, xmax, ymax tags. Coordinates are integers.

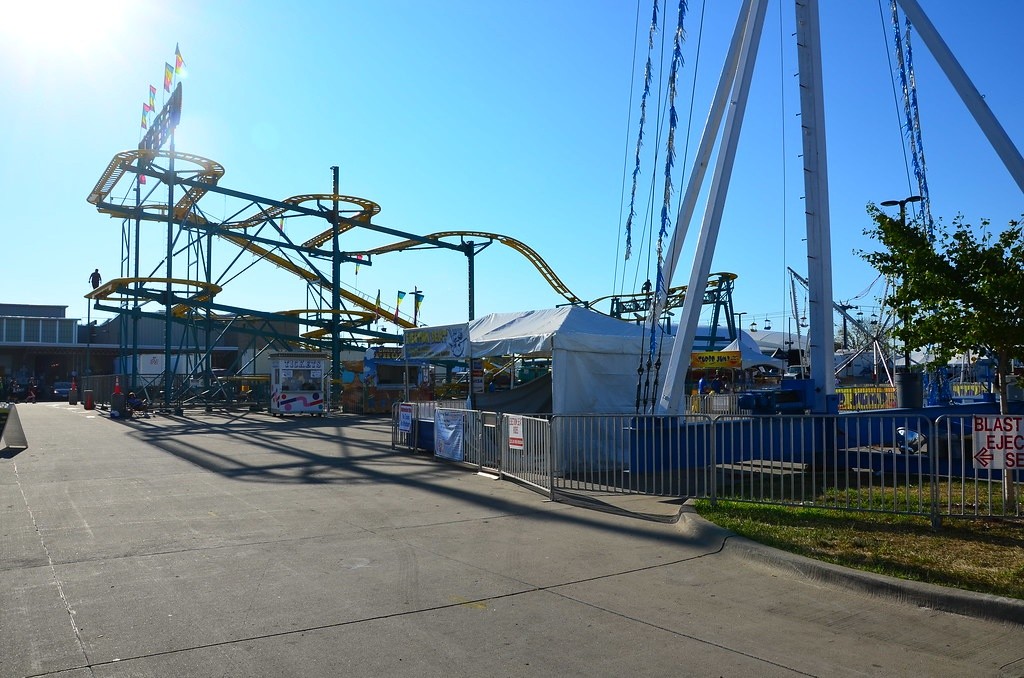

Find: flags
<box><xmin>355</xmin><ymin>255</ymin><xmax>363</xmax><ymax>275</ymax></box>
<box><xmin>141</xmin><ymin>103</ymin><xmax>150</xmax><ymax>130</ymax></box>
<box><xmin>279</xmin><ymin>214</ymin><xmax>284</xmax><ymax>231</ymax></box>
<box><xmin>164</xmin><ymin>62</ymin><xmax>174</xmax><ymax>94</ymax></box>
<box><xmin>139</xmin><ymin>174</ymin><xmax>146</xmax><ymax>185</ymax></box>
<box><xmin>374</xmin><ymin>289</ymin><xmax>381</xmax><ymax>325</ymax></box>
<box><xmin>175</xmin><ymin>46</ymin><xmax>184</xmax><ymax>75</ymax></box>
<box><xmin>415</xmin><ymin>294</ymin><xmax>425</xmax><ymax>316</ymax></box>
<box><xmin>394</xmin><ymin>291</ymin><xmax>406</xmax><ymax>324</ymax></box>
<box><xmin>149</xmin><ymin>84</ymin><xmax>156</xmax><ymax>113</ymax></box>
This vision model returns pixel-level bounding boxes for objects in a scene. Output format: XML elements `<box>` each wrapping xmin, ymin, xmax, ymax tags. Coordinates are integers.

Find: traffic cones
<box><xmin>86</xmin><ymin>393</ymin><xmax>92</xmax><ymax>410</ymax></box>
<box><xmin>72</xmin><ymin>377</ymin><xmax>76</xmax><ymax>390</ymax></box>
<box><xmin>115</xmin><ymin>375</ymin><xmax>121</xmax><ymax>394</ymax></box>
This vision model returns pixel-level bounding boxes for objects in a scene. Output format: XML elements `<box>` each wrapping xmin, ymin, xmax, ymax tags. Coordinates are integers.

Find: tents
<box><xmin>720</xmin><ymin>337</ymin><xmax>789</xmax><ymax>386</ymax></box>
<box><xmin>896</xmin><ymin>338</ymin><xmax>996</xmax><ymax>377</ymax></box>
<box><xmin>466</xmin><ymin>305</ymin><xmax>687</xmax><ymax>479</ymax></box>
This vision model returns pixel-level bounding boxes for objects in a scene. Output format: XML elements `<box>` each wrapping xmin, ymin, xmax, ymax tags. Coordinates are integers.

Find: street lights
<box><xmin>881</xmin><ymin>196</ymin><xmax>925</xmax><ymax>368</ymax></box>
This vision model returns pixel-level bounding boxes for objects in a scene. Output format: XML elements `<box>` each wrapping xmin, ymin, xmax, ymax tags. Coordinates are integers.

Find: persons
<box><xmin>282</xmin><ymin>371</ymin><xmax>316</xmax><ymax>390</ymax></box>
<box><xmin>834</xmin><ymin>375</ymin><xmax>842</xmax><ymax>388</ymax></box>
<box><xmin>722</xmin><ymin>376</ymin><xmax>732</xmax><ymax>414</ymax></box>
<box><xmin>698</xmin><ymin>373</ymin><xmax>712</xmax><ymax>414</ymax></box>
<box><xmin>442</xmin><ymin>375</ymin><xmax>463</xmax><ymax>386</ymax></box>
<box><xmin>712</xmin><ymin>374</ymin><xmax>723</xmax><ymax>397</ymax></box>
<box><xmin>641</xmin><ymin>278</ymin><xmax>653</xmax><ymax>299</ymax></box>
<box><xmin>6</xmin><ymin>375</ymin><xmax>38</xmax><ymax>403</ymax></box>
<box><xmin>489</xmin><ymin>378</ymin><xmax>496</xmax><ymax>392</ymax></box>
<box><xmin>88</xmin><ymin>269</ymin><xmax>102</xmax><ymax>290</ymax></box>
<box><xmin>127</xmin><ymin>391</ymin><xmax>151</xmax><ymax>419</ymax></box>
